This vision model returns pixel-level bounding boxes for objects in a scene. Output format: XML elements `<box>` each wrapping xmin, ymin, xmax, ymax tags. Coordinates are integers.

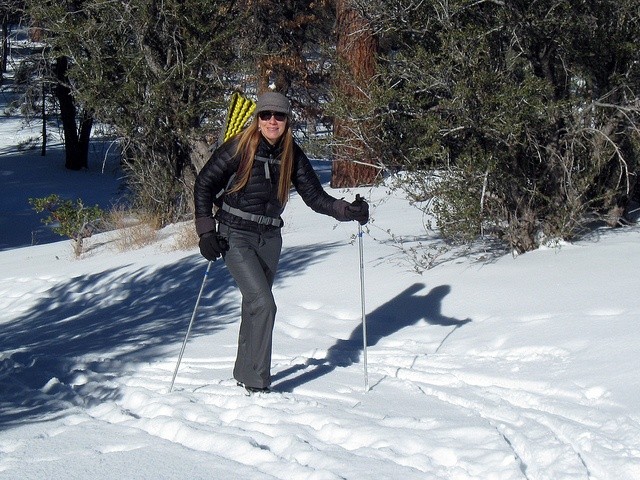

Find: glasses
<box><xmin>259</xmin><ymin>111</ymin><xmax>286</xmax><ymax>121</ymax></box>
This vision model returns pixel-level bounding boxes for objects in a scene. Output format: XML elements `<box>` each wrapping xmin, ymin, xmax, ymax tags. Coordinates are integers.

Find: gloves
<box><xmin>332</xmin><ymin>198</ymin><xmax>370</xmax><ymax>226</ymax></box>
<box><xmin>195</xmin><ymin>216</ymin><xmax>227</xmax><ymax>262</ymax></box>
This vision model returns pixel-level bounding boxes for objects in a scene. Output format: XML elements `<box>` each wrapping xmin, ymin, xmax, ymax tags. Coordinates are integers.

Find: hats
<box><xmin>254</xmin><ymin>92</ymin><xmax>292</xmax><ymax>117</ymax></box>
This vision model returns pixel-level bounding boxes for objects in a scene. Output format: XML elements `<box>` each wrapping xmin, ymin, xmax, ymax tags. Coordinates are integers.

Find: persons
<box><xmin>193</xmin><ymin>92</ymin><xmax>368</xmax><ymax>391</ymax></box>
